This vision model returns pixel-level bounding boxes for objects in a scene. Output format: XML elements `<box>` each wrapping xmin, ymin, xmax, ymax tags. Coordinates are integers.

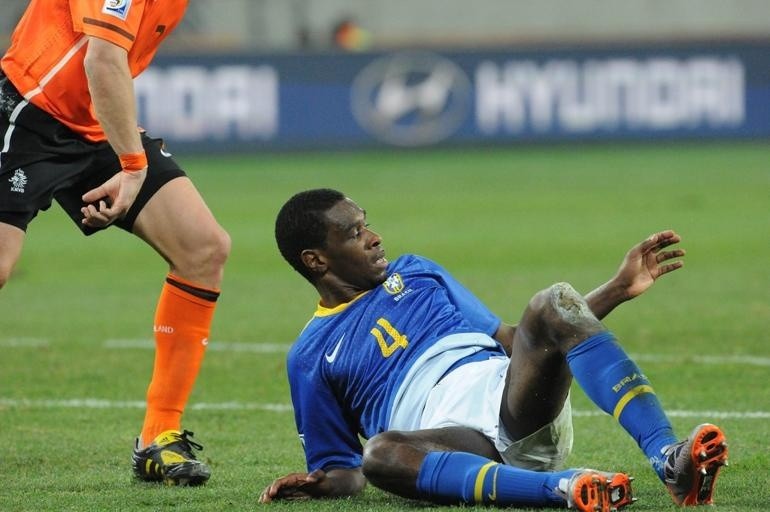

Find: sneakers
<box><xmin>131</xmin><ymin>430</ymin><xmax>212</xmax><ymax>486</ymax></box>
<box><xmin>565</xmin><ymin>470</ymin><xmax>638</xmax><ymax>511</ymax></box>
<box><xmin>662</xmin><ymin>422</ymin><xmax>729</xmax><ymax>508</ymax></box>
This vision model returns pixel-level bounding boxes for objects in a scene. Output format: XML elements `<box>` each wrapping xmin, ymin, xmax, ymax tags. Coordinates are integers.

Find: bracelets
<box><xmin>118</xmin><ymin>150</ymin><xmax>149</xmax><ymax>173</ymax></box>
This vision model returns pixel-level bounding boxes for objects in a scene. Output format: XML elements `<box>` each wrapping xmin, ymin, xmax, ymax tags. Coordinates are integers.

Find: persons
<box><xmin>1</xmin><ymin>1</ymin><xmax>236</xmax><ymax>487</ymax></box>
<box><xmin>253</xmin><ymin>188</ymin><xmax>730</xmax><ymax>512</ymax></box>
